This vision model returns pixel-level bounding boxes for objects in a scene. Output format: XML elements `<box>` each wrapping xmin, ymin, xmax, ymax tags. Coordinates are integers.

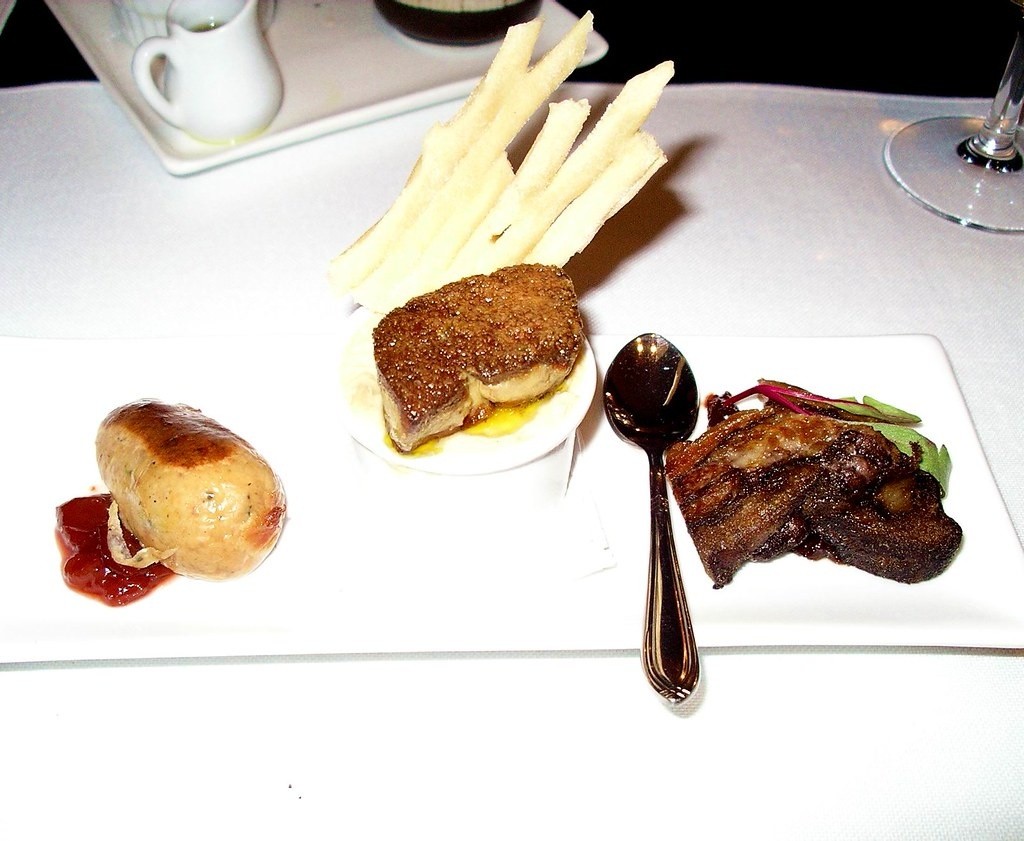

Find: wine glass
<box><xmin>883</xmin><ymin>1</ymin><xmax>1024</xmax><ymax>235</ymax></box>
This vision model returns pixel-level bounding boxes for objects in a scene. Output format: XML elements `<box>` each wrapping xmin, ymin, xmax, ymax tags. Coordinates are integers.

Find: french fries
<box><xmin>334</xmin><ymin>12</ymin><xmax>674</xmax><ymax>314</ymax></box>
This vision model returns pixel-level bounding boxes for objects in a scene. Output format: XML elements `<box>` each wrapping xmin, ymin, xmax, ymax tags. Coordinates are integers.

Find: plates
<box><xmin>373</xmin><ymin>0</ymin><xmax>543</xmax><ymax>46</ymax></box>
<box><xmin>49</xmin><ymin>0</ymin><xmax>609</xmax><ymax>177</ymax></box>
<box><xmin>323</xmin><ymin>305</ymin><xmax>597</xmax><ymax>477</ymax></box>
<box><xmin>1</xmin><ymin>333</ymin><xmax>1024</xmax><ymax>680</ymax></box>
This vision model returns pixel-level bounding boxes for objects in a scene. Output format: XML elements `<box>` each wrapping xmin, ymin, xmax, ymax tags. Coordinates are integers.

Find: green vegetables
<box><xmin>718</xmin><ymin>382</ymin><xmax>952</xmax><ymax>499</ymax></box>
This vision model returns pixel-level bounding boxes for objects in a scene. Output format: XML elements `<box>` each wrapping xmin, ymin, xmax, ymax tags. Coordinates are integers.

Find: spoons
<box><xmin>601</xmin><ymin>333</ymin><xmax>701</xmax><ymax>710</ymax></box>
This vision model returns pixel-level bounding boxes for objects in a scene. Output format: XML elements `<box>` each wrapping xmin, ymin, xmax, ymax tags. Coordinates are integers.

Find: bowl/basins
<box><xmin>111</xmin><ymin>0</ymin><xmax>276</xmax><ymax>58</ymax></box>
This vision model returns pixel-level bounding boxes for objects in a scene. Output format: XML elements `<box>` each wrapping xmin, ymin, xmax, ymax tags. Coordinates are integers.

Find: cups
<box><xmin>131</xmin><ymin>0</ymin><xmax>283</xmax><ymax>146</ymax></box>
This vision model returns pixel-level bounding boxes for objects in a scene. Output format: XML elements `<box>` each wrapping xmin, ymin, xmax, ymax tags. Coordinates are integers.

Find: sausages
<box><xmin>94</xmin><ymin>399</ymin><xmax>285</xmax><ymax>581</ymax></box>
